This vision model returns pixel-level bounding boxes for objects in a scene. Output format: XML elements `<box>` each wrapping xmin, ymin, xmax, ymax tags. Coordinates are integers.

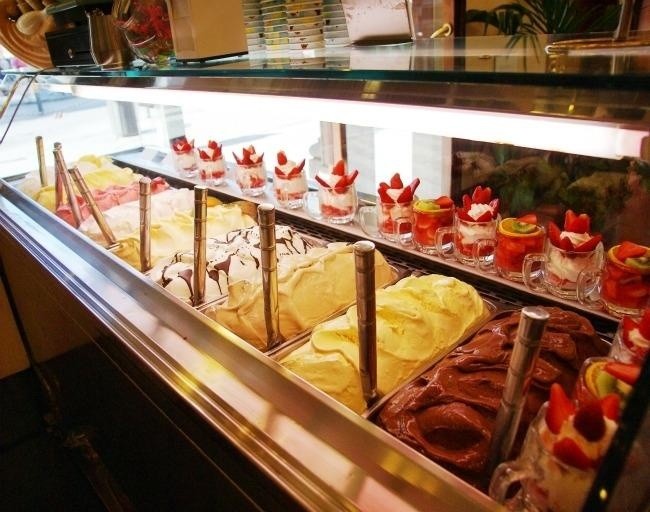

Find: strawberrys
<box><xmin>197</xmin><ymin>140</ymin><xmax>225</xmax><ymax>179</ymax></box>
<box><xmin>232</xmin><ymin>144</ymin><xmax>266</xmax><ymax>189</ymax></box>
<box><xmin>174</xmin><ymin>140</ymin><xmax>191</xmax><ymax>155</ymax></box>
<box><xmin>315</xmin><ymin>160</ymin><xmax>359</xmax><ymax>216</ymax></box>
<box><xmin>378</xmin><ymin>172</ymin><xmax>650</xmax><ymax>470</ymax></box>
<box><xmin>274</xmin><ymin>150</ymin><xmax>306</xmax><ymax>200</ymax></box>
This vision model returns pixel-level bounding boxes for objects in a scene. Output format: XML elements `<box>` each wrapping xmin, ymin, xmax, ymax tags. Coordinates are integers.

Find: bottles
<box><xmin>110</xmin><ymin>0</ymin><xmax>175</xmax><ymax>61</ymax></box>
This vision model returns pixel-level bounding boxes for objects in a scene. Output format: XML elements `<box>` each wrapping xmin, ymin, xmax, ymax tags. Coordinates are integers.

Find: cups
<box><xmin>521</xmin><ymin>236</ymin><xmax>604</xmax><ymax>302</ymax></box>
<box><xmin>575</xmin><ymin>244</ymin><xmax>649</xmax><ymax>319</ymax></box>
<box><xmin>303</xmin><ymin>177</ymin><xmax>358</xmax><ymax>225</ymax></box>
<box><xmin>272</xmin><ymin>169</ymin><xmax>308</xmax><ymax>211</ymax></box>
<box><xmin>393</xmin><ymin>197</ymin><xmax>456</xmax><ymax>256</ymax></box>
<box><xmin>170</xmin><ymin>145</ymin><xmax>226</xmax><ymax>186</ymax></box>
<box><xmin>232</xmin><ymin>160</ymin><xmax>268</xmax><ymax>197</ymax></box>
<box><xmin>359</xmin><ymin>194</ymin><xmax>420</xmax><ymax>243</ymax></box>
<box><xmin>487</xmin><ymin>316</ymin><xmax>648</xmax><ymax>511</ymax></box>
<box><xmin>472</xmin><ymin>217</ymin><xmax>545</xmax><ymax>283</ymax></box>
<box><xmin>434</xmin><ymin>212</ymin><xmax>502</xmax><ymax>267</ymax></box>
